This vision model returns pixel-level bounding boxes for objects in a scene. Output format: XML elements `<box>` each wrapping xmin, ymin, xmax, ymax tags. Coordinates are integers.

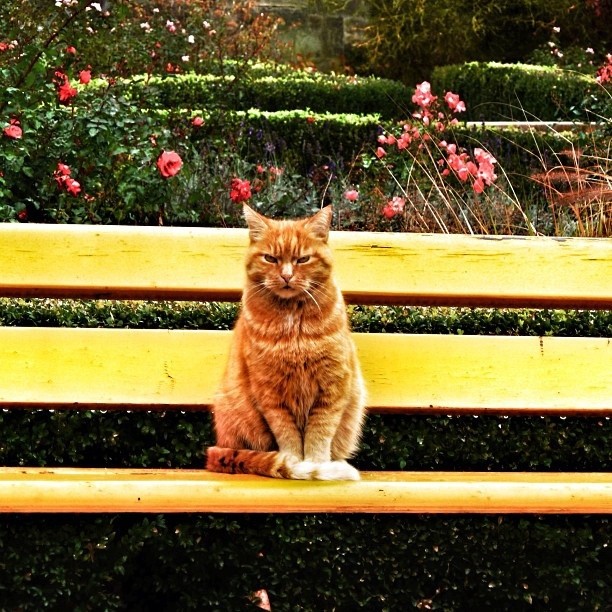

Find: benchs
<box><xmin>0</xmin><ymin>222</ymin><xmax>612</xmax><ymax>512</ymax></box>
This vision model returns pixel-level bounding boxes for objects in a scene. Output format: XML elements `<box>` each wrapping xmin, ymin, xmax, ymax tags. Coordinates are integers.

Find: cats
<box><xmin>206</xmin><ymin>202</ymin><xmax>367</xmax><ymax>480</ymax></box>
